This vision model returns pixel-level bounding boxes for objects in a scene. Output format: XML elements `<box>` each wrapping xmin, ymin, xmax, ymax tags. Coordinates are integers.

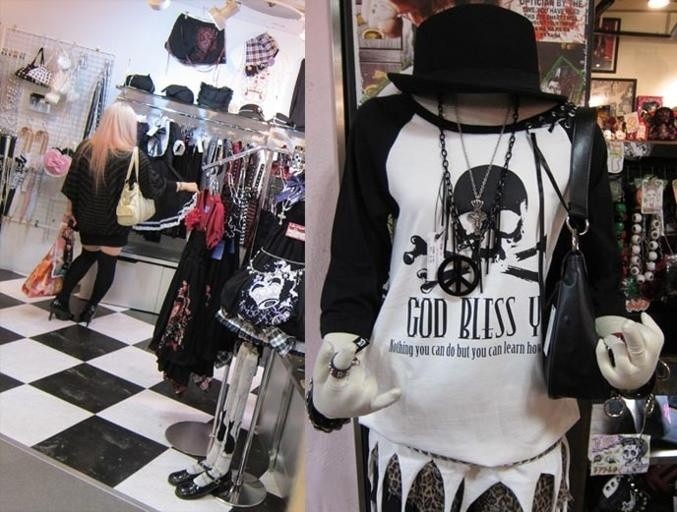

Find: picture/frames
<box><xmin>588</xmin><ymin>78</ymin><xmax>637</xmax><ymax>117</ymax></box>
<box><xmin>590</xmin><ymin>17</ymin><xmax>621</xmax><ymax>73</ymax></box>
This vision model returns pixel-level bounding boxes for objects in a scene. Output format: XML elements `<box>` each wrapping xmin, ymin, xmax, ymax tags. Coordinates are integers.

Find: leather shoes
<box><xmin>167</xmin><ymin>458</ymin><xmax>232</xmax><ymax>500</ymax></box>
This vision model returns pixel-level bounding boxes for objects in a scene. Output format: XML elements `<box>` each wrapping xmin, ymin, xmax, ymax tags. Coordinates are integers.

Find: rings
<box><xmin>329</xmin><ymin>351</ymin><xmax>355</xmax><ymax>379</ymax></box>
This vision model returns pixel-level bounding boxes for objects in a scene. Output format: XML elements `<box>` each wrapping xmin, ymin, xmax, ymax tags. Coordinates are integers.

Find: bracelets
<box><xmin>177</xmin><ymin>181</ymin><xmax>183</xmax><ymax>191</ymax></box>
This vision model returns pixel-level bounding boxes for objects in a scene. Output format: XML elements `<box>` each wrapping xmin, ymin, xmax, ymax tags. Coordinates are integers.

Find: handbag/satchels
<box><xmin>543</xmin><ymin>230</ymin><xmax>610</xmax><ymax>402</ymax></box>
<box><xmin>197</xmin><ymin>81</ymin><xmax>233</xmax><ymax>112</ymax></box>
<box><xmin>162</xmin><ymin>85</ymin><xmax>194</xmax><ymax>104</ymax></box>
<box><xmin>116</xmin><ymin>146</ymin><xmax>156</xmax><ymax>226</ymax></box>
<box><xmin>22</xmin><ymin>222</ymin><xmax>80</xmax><ymax>298</ymax></box>
<box><xmin>120</xmin><ymin>73</ymin><xmax>155</xmax><ymax>94</ymax></box>
<box><xmin>41</xmin><ymin>145</ymin><xmax>75</xmax><ymax>177</ymax></box>
<box><xmin>14</xmin><ymin>47</ymin><xmax>57</xmax><ymax>88</ymax></box>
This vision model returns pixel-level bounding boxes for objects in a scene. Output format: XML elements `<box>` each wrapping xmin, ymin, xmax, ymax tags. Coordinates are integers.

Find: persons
<box><xmin>48</xmin><ymin>101</ymin><xmax>200</xmax><ymax>328</ymax></box>
<box><xmin>304</xmin><ymin>4</ymin><xmax>667</xmax><ymax>512</ymax></box>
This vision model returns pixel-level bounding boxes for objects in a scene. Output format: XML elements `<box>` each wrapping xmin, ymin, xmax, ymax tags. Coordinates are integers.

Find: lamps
<box><xmin>207</xmin><ymin>0</ymin><xmax>241</xmax><ymax>31</ymax></box>
<box><xmin>148</xmin><ymin>0</ymin><xmax>171</xmax><ymax>12</ymax></box>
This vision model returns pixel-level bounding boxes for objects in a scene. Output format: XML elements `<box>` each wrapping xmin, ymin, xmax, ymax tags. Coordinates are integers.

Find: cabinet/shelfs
<box><xmin>79</xmin><ymin>87</ymin><xmax>305</xmax><ymax>316</ymax></box>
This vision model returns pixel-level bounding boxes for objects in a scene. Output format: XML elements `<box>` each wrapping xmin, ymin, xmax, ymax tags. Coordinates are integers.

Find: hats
<box><xmin>386</xmin><ymin>4</ymin><xmax>568</xmax><ymax>101</ymax></box>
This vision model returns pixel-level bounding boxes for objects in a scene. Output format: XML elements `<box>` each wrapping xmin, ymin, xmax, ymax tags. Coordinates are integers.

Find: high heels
<box><xmin>49</xmin><ymin>299</ymin><xmax>75</xmax><ymax>320</ymax></box>
<box><xmin>80</xmin><ymin>304</ymin><xmax>96</xmax><ymax>329</ymax></box>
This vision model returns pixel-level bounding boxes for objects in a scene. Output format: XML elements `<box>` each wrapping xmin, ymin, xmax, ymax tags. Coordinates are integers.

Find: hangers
<box><xmin>206</xmin><ymin>145</ymin><xmax>266</xmax><ymax>193</ymax></box>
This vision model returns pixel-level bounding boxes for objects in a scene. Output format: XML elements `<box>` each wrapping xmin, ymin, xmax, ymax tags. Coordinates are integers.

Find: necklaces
<box><xmin>436</xmin><ymin>106</ymin><xmax>521</xmax><ymax>293</ymax></box>
